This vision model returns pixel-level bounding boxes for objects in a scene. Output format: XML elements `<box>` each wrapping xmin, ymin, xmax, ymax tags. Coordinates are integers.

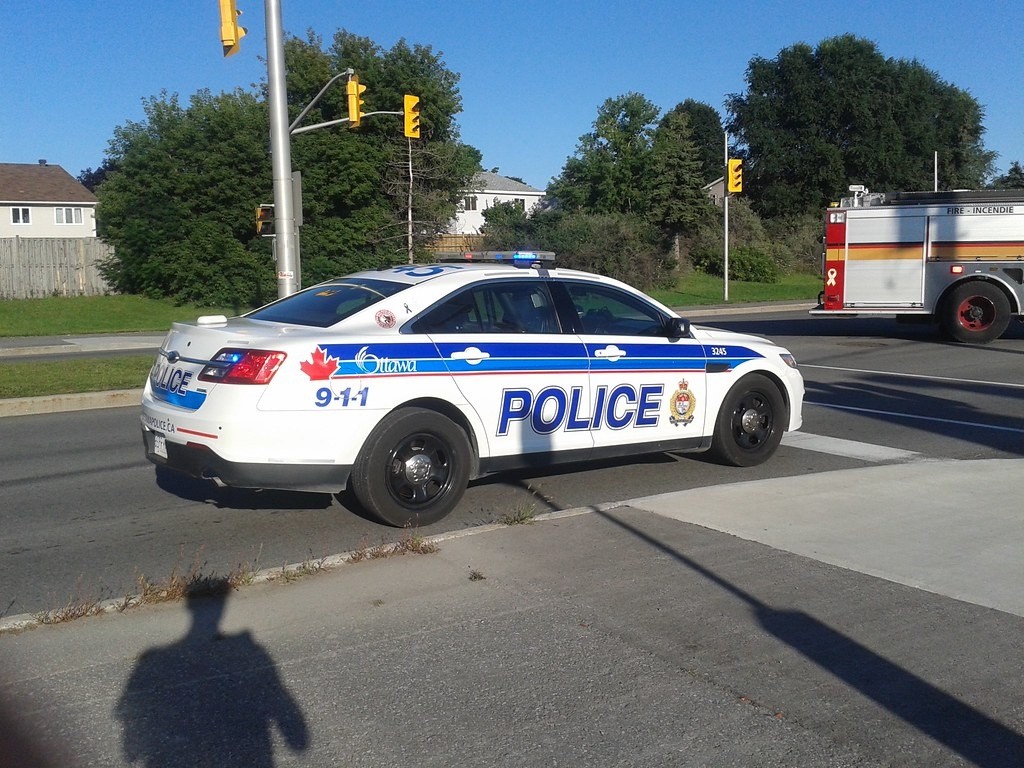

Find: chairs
<box><xmin>502</xmin><ymin>293</ymin><xmax>535</xmax><ymax>329</ymax></box>
<box><xmin>442</xmin><ymin>292</ymin><xmax>481</xmax><ymax>332</ymax></box>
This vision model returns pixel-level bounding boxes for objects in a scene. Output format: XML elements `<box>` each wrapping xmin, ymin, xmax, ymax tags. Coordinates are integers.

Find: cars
<box><xmin>140</xmin><ymin>250</ymin><xmax>805</xmax><ymax>527</ymax></box>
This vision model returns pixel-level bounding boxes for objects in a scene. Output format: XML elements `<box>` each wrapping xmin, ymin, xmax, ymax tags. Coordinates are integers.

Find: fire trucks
<box><xmin>808</xmin><ymin>185</ymin><xmax>1024</xmax><ymax>345</ymax></box>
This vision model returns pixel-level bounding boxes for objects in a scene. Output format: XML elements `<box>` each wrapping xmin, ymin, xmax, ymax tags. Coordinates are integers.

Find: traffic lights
<box><xmin>256</xmin><ymin>207</ymin><xmax>272</xmax><ymax>233</ymax></box>
<box><xmin>404</xmin><ymin>95</ymin><xmax>424</xmax><ymax>138</ymax></box>
<box><xmin>346</xmin><ymin>76</ymin><xmax>367</xmax><ymax>130</ymax></box>
<box><xmin>728</xmin><ymin>159</ymin><xmax>743</xmax><ymax>194</ymax></box>
<box><xmin>219</xmin><ymin>0</ymin><xmax>248</xmax><ymax>57</ymax></box>
